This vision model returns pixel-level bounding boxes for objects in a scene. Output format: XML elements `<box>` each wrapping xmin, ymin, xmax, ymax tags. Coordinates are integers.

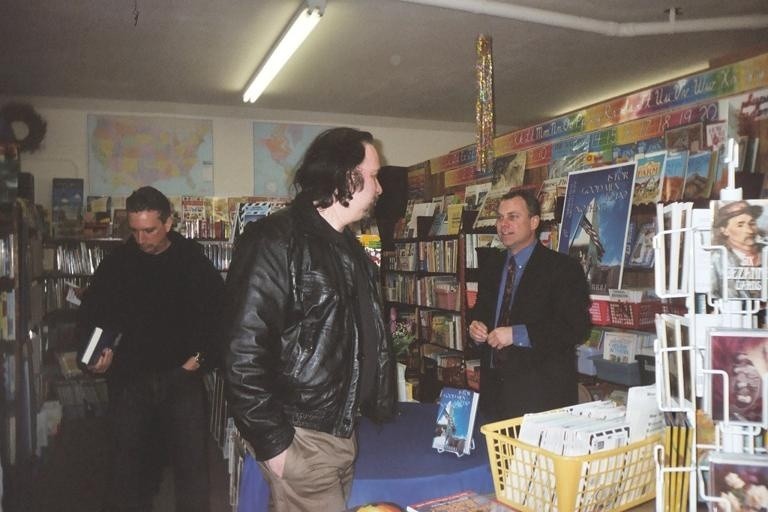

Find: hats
<box><xmin>712</xmin><ymin>200</ymin><xmax>763</xmax><ymax>228</ymax></box>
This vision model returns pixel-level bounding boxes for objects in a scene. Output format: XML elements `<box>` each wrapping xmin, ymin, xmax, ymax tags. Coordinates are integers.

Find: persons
<box><xmin>72</xmin><ymin>184</ymin><xmax>232</xmax><ymax>511</ymax></box>
<box><xmin>220</xmin><ymin>124</ymin><xmax>398</xmax><ymax>512</ymax></box>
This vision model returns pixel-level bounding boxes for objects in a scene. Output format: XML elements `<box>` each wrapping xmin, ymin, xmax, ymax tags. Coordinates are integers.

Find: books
<box><xmin>236</xmin><ymin>198</ymin><xmax>291</xmax><ymax>234</ymax></box>
<box><xmin>406</xmin><ymin>489</ymin><xmax>522</xmax><ymax>512</ymax></box>
<box><xmin>34</xmin><ymin>188</ymin><xmax>236</xmax><ymax>414</ymax></box>
<box><xmin>471</xmin><ymin>95</ymin><xmax>768</xmax><ymax>511</ymax></box>
<box><xmin>199</xmin><ymin>368</ymin><xmax>245</xmax><ymax>512</ymax></box>
<box><xmin>431</xmin><ymin>385</ymin><xmax>481</xmax><ymax>457</ymax></box>
<box><xmin>1</xmin><ymin>231</ymin><xmax>33</xmax><ymax>474</ymax></box>
<box><xmin>352</xmin><ymin>181</ymin><xmax>470</xmax><ymax>393</ymax></box>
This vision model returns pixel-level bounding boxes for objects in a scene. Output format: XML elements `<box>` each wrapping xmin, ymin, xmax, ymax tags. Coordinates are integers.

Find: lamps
<box><xmin>244</xmin><ymin>0</ymin><xmax>329</xmax><ymax>104</ymax></box>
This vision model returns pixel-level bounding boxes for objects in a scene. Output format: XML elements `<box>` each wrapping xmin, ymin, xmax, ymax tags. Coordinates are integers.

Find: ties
<box><xmin>489</xmin><ymin>255</ymin><xmax>518</xmax><ymax>364</ymax></box>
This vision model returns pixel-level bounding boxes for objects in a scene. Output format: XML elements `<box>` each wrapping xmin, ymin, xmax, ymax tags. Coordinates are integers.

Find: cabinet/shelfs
<box><xmin>0</xmin><ymin>144</ymin><xmax>768</xmax><ymax>512</ymax></box>
